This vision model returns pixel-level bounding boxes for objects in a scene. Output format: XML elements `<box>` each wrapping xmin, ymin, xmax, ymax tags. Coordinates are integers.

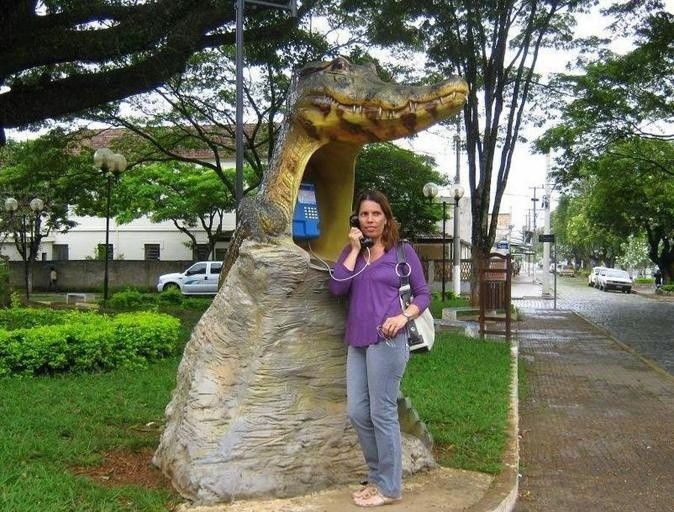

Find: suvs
<box><xmin>155</xmin><ymin>260</ymin><xmax>222</xmax><ymax>299</ymax></box>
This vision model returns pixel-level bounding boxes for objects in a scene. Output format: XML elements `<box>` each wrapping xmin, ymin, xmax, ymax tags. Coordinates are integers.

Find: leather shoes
<box><xmin>351</xmin><ymin>482</ymin><xmax>403</xmax><ymax>507</ymax></box>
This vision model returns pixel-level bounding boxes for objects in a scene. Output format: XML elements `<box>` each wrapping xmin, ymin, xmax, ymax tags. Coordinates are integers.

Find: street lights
<box><xmin>91</xmin><ymin>146</ymin><xmax>125</xmax><ymax>302</ymax></box>
<box><xmin>419</xmin><ymin>183</ymin><xmax>465</xmax><ymax>304</ymax></box>
<box><xmin>4</xmin><ymin>193</ymin><xmax>44</xmax><ymax>305</ymax></box>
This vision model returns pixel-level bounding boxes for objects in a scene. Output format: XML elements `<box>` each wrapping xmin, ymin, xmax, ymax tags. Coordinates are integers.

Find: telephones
<box><xmin>349</xmin><ymin>215</ymin><xmax>374</xmax><ymax>248</ymax></box>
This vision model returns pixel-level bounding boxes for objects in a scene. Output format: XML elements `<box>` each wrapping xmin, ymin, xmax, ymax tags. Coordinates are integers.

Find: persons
<box><xmin>48</xmin><ymin>267</ymin><xmax>60</xmax><ymax>292</ymax></box>
<box><xmin>327</xmin><ymin>189</ymin><xmax>433</xmax><ymax>507</ymax></box>
<box><xmin>653</xmin><ymin>269</ymin><xmax>662</xmax><ymax>290</ymax></box>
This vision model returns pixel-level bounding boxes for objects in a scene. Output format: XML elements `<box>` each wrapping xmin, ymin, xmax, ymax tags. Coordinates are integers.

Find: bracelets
<box><xmin>403</xmin><ymin>310</ymin><xmax>412</xmax><ymax>322</ymax></box>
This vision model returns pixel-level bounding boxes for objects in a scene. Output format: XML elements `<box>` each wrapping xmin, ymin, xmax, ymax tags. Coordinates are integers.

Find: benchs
<box><xmin>442</xmin><ymin>306</ymin><xmax>497</xmax><ymax>323</ymax></box>
<box><xmin>66</xmin><ymin>292</ymin><xmax>95</xmax><ymax>305</ymax></box>
<box><xmin>434</xmin><ymin>318</ymin><xmax>487</xmax><ymax>340</ymax></box>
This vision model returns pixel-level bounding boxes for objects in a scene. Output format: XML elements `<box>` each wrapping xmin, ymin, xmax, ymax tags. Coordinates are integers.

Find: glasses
<box><xmin>376</xmin><ymin>323</ymin><xmax>396</xmax><ymax>349</ymax></box>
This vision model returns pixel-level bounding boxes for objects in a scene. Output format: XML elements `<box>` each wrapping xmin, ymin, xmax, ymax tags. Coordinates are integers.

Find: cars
<box><xmin>536</xmin><ymin>261</ymin><xmax>574</xmax><ymax>277</ymax></box>
<box><xmin>588</xmin><ymin>265</ymin><xmax>606</xmax><ymax>286</ymax></box>
<box><xmin>595</xmin><ymin>268</ymin><xmax>632</xmax><ymax>294</ymax></box>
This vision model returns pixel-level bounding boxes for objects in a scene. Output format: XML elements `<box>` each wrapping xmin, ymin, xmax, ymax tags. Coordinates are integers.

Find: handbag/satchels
<box><xmin>398</xmin><ymin>283</ymin><xmax>436</xmax><ymax>355</ymax></box>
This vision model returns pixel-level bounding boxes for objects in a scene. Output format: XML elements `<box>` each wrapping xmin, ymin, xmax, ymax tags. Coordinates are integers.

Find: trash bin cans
<box><xmin>479</xmin><ymin>253</ymin><xmax>512</xmax><ymax>342</ymax></box>
